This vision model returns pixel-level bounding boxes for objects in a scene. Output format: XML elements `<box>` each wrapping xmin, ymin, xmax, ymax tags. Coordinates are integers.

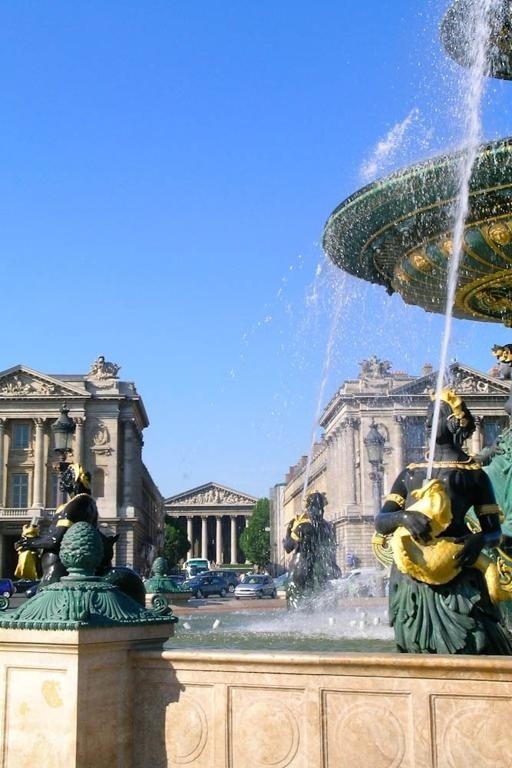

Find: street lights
<box><xmin>361</xmin><ymin>416</ymin><xmax>391</xmax><ymax>522</ymax></box>
<box><xmin>49</xmin><ymin>399</ymin><xmax>90</xmax><ymax>507</ymax></box>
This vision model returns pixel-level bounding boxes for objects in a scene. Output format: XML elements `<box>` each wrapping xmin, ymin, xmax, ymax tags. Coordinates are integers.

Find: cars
<box><xmin>327</xmin><ymin>566</ymin><xmax>384</xmax><ymax>595</ymax></box>
<box><xmin>232</xmin><ymin>574</ymin><xmax>278</xmax><ymax>600</ymax></box>
<box><xmin>0</xmin><ymin>577</ymin><xmax>17</xmax><ymax>599</ymax></box>
<box><xmin>196</xmin><ymin>570</ymin><xmax>238</xmax><ymax>592</ymax></box>
<box><xmin>12</xmin><ymin>576</ymin><xmax>41</xmax><ymax>594</ymax></box>
<box><xmin>181</xmin><ymin>575</ymin><xmax>229</xmax><ymax>598</ymax></box>
<box><xmin>272</xmin><ymin>571</ymin><xmax>290</xmax><ymax>591</ymax></box>
<box><xmin>168</xmin><ymin>574</ymin><xmax>186</xmax><ymax>587</ymax></box>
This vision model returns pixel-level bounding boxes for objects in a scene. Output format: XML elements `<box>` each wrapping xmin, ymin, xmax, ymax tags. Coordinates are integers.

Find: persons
<box><xmin>280</xmin><ymin>490</ymin><xmax>343</xmax><ymax>608</ymax></box>
<box><xmin>372</xmin><ymin>386</ymin><xmax>510</xmax><ymax>653</ymax></box>
<box><xmin>139</xmin><ymin>564</ymin><xmax>152</xmax><ymax>583</ymax></box>
<box><xmin>13</xmin><ymin>461</ymin><xmax>100</xmax><ymax>590</ymax></box>
<box><xmin>237</xmin><ymin>565</ymin><xmax>273</xmax><ymax>586</ymax></box>
<box><xmin>203</xmin><ymin>579</ymin><xmax>209</xmax><ymax>584</ymax></box>
<box><xmin>466</xmin><ymin>342</ymin><xmax>512</xmax><ymax>529</ymax></box>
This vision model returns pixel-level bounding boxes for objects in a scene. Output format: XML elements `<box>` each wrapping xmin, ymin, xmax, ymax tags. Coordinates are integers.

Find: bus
<box><xmin>182</xmin><ymin>558</ymin><xmax>212</xmax><ymax>579</ymax></box>
